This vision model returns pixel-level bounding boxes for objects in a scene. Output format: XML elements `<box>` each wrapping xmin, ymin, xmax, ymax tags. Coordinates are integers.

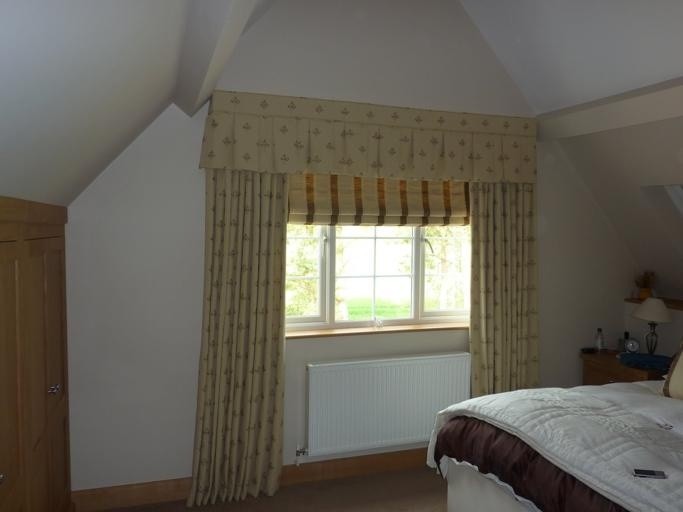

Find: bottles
<box><xmin>594</xmin><ymin>327</ymin><xmax>604</xmax><ymax>350</ymax></box>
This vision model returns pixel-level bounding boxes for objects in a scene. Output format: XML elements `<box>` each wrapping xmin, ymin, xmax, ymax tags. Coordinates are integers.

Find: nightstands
<box><xmin>579</xmin><ymin>349</ymin><xmax>662</xmax><ymax>386</ymax></box>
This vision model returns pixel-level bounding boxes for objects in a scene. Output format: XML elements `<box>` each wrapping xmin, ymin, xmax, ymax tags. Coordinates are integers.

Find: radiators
<box><xmin>303</xmin><ymin>351</ymin><xmax>473</xmax><ymax>458</ymax></box>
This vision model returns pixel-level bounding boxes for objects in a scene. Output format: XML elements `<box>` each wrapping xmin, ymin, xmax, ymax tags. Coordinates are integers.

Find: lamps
<box><xmin>630</xmin><ymin>297</ymin><xmax>672</xmax><ymax>354</ymax></box>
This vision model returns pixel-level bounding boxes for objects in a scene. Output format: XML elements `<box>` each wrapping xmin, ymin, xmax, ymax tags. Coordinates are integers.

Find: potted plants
<box><xmin>634</xmin><ymin>269</ymin><xmax>657</xmax><ymax>300</ymax></box>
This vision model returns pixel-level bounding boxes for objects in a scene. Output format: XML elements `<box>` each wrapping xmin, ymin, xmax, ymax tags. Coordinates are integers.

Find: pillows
<box><xmin>661</xmin><ymin>349</ymin><xmax>682</xmax><ymax>397</ymax></box>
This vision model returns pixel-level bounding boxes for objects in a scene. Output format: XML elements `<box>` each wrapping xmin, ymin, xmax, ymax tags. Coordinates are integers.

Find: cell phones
<box><xmin>633</xmin><ymin>469</ymin><xmax>665</xmax><ymax>479</ymax></box>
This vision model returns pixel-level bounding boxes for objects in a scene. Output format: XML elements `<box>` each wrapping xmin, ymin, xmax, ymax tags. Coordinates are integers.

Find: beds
<box><xmin>431</xmin><ymin>375</ymin><xmax>682</xmax><ymax>511</ymax></box>
